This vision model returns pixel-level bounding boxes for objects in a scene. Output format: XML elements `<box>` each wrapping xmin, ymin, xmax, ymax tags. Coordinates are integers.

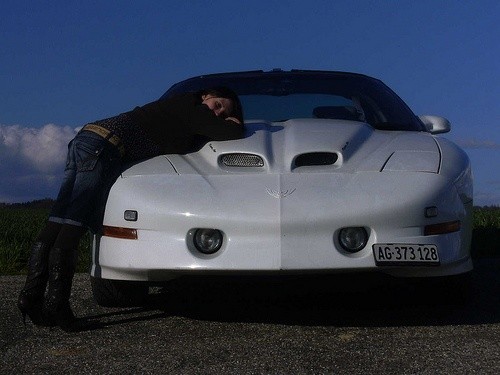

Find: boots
<box><xmin>18</xmin><ymin>240</ymin><xmax>50</xmax><ymax>327</ymax></box>
<box><xmin>39</xmin><ymin>246</ymin><xmax>87</xmax><ymax>332</ymax></box>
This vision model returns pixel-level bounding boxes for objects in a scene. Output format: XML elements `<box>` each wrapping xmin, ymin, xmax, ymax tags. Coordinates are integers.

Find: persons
<box><xmin>18</xmin><ymin>80</ymin><xmax>252</xmax><ymax>332</ymax></box>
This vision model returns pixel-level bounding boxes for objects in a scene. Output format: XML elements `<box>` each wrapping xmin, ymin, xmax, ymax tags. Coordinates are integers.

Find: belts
<box><xmin>83</xmin><ymin>123</ymin><xmax>120</xmax><ymax>147</ymax></box>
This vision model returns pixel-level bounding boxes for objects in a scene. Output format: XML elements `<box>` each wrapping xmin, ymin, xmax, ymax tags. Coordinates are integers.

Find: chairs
<box><xmin>313</xmin><ymin>104</ymin><xmax>362</xmax><ymax>123</ymax></box>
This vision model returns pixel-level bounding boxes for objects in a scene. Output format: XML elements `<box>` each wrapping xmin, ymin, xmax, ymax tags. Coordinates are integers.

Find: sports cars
<box><xmin>89</xmin><ymin>67</ymin><xmax>476</xmax><ymax>308</ymax></box>
